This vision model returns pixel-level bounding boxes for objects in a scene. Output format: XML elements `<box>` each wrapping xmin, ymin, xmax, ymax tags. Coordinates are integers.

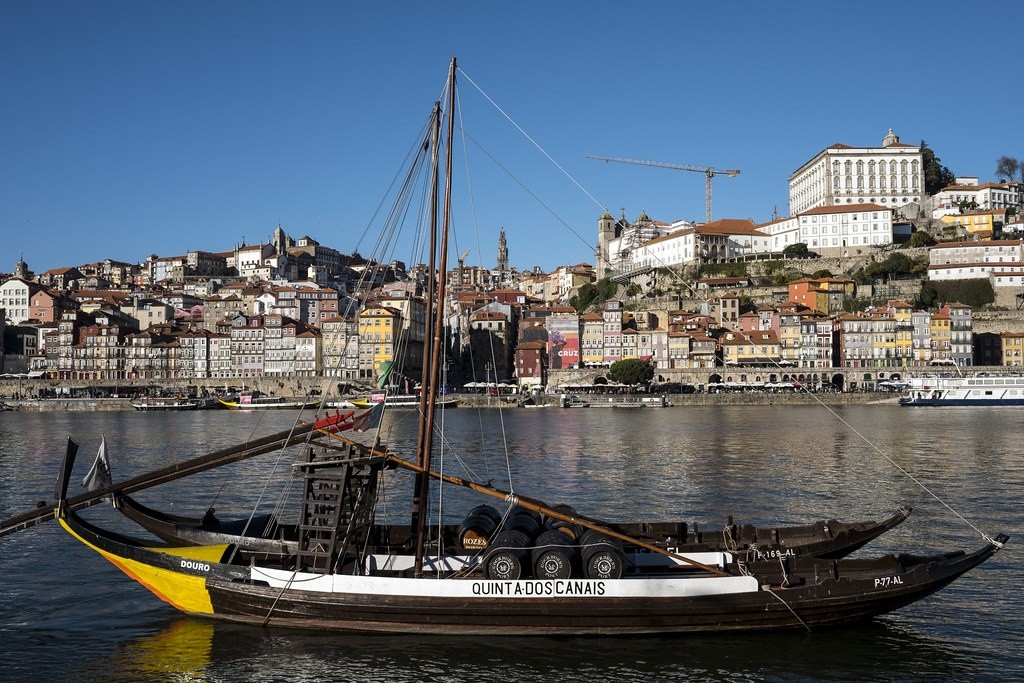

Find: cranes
<box><xmin>584</xmin><ymin>155</ymin><xmax>741</xmax><ymax>224</ymax></box>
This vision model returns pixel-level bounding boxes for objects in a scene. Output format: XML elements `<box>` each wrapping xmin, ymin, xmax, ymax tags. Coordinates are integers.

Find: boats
<box><xmin>218</xmin><ymin>396</ymin><xmax>324</xmax><ymax>411</ymax></box>
<box><xmin>348</xmin><ymin>394</ymin><xmax>462</xmax><ymax>409</ymax></box>
<box><xmin>131</xmin><ymin>396</ymin><xmax>198</xmax><ymax>411</ymax></box>
<box><xmin>898</xmin><ymin>377</ymin><xmax>1024</xmax><ymax>406</ymax></box>
<box><xmin>564</xmin><ymin>396</ymin><xmax>590</xmax><ymax>407</ymax></box>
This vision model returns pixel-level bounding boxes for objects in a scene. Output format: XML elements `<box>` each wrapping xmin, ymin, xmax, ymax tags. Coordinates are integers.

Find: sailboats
<box><xmin>84</xmin><ymin>101</ymin><xmax>913</xmax><ymax>561</ymax></box>
<box><xmin>55</xmin><ymin>58</ymin><xmax>1011</xmax><ymax>637</ymax></box>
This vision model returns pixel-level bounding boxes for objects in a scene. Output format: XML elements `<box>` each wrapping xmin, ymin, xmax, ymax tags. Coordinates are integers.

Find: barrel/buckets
<box><xmin>458</xmin><ymin>503</ymin><xmax>625</xmax><ymax>579</ymax></box>
